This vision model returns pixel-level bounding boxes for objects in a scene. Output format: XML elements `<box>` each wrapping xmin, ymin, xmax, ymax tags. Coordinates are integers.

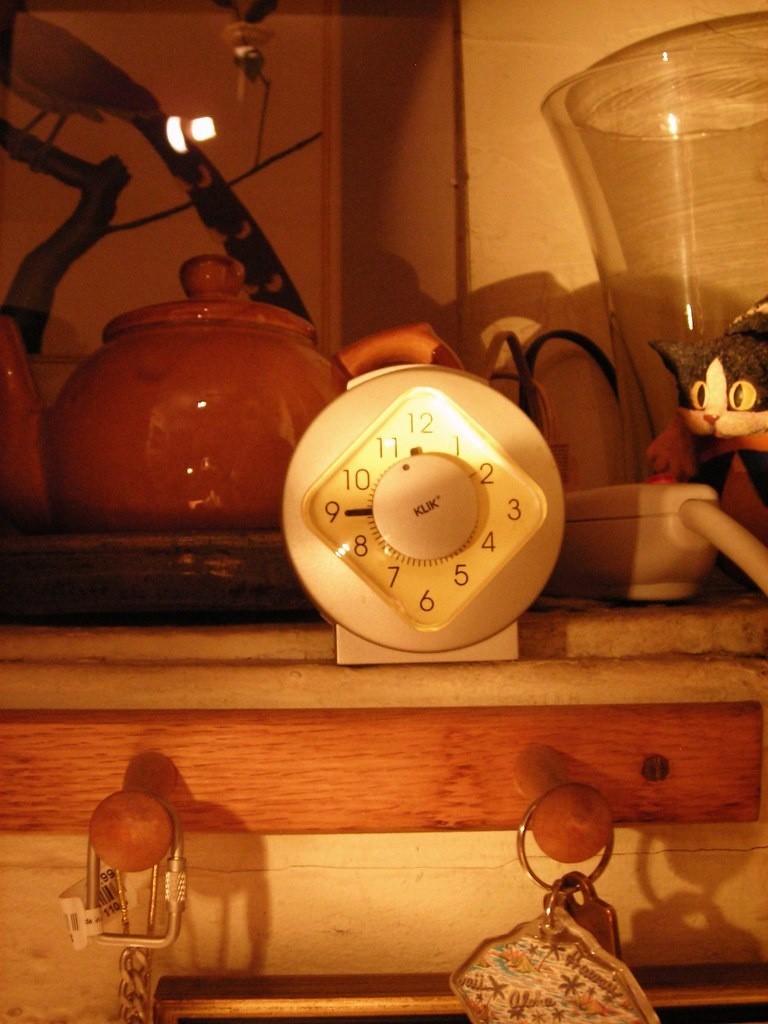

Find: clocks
<box><xmin>282</xmin><ymin>363</ymin><xmax>568</xmax><ymax>668</ymax></box>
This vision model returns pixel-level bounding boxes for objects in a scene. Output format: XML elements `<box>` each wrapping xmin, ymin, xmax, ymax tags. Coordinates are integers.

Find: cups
<box><xmin>557</xmin><ymin>484</ymin><xmax>719</xmax><ymax>600</ymax></box>
<box><xmin>538</xmin><ymin>44</ymin><xmax>767</xmax><ymax>535</ymax></box>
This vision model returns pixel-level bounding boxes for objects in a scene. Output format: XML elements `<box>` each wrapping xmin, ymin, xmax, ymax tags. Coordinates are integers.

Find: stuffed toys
<box><xmin>647</xmin><ymin>312</ymin><xmax>768</xmax><ymax>547</ymax></box>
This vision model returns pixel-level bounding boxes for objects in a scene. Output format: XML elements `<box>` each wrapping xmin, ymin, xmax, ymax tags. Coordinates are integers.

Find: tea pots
<box><xmin>0</xmin><ymin>253</ymin><xmax>463</xmax><ymax>532</ymax></box>
